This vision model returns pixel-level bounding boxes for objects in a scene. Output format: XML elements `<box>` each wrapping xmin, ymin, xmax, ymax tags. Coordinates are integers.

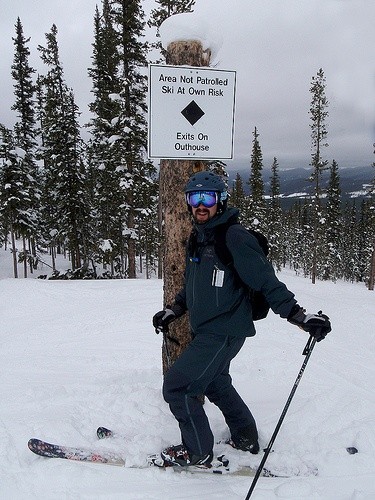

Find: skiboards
<box><xmin>28</xmin><ymin>426</ymin><xmax>359</xmax><ymax>479</ymax></box>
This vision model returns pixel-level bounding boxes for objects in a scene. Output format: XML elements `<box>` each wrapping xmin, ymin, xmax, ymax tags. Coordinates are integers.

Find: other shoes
<box><xmin>216</xmin><ymin>438</ymin><xmax>260</xmax><ymax>454</ymax></box>
<box><xmin>151</xmin><ymin>445</ymin><xmax>214</xmax><ymax>468</ymax></box>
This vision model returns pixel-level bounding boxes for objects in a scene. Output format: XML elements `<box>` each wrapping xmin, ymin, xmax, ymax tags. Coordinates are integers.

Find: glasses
<box><xmin>189</xmin><ymin>191</ymin><xmax>218</xmax><ymax>208</ymax></box>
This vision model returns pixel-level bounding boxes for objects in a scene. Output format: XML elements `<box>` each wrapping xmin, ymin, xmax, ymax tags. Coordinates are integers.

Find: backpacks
<box><xmin>246</xmin><ymin>230</ymin><xmax>270</xmax><ymax>320</ymax></box>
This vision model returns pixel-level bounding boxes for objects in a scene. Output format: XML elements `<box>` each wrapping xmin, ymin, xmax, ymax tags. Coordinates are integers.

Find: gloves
<box><xmin>152</xmin><ymin>309</ymin><xmax>176</xmax><ymax>334</ymax></box>
<box><xmin>301</xmin><ymin>313</ymin><xmax>331</xmax><ymax>342</ymax></box>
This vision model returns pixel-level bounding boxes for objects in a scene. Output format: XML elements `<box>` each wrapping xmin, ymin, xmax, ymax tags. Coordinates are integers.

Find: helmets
<box><xmin>184</xmin><ymin>171</ymin><xmax>225</xmax><ymax>193</ymax></box>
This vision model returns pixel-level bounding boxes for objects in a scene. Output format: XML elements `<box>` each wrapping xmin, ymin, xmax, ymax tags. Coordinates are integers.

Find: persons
<box><xmin>147</xmin><ymin>171</ymin><xmax>332</xmax><ymax>467</ymax></box>
